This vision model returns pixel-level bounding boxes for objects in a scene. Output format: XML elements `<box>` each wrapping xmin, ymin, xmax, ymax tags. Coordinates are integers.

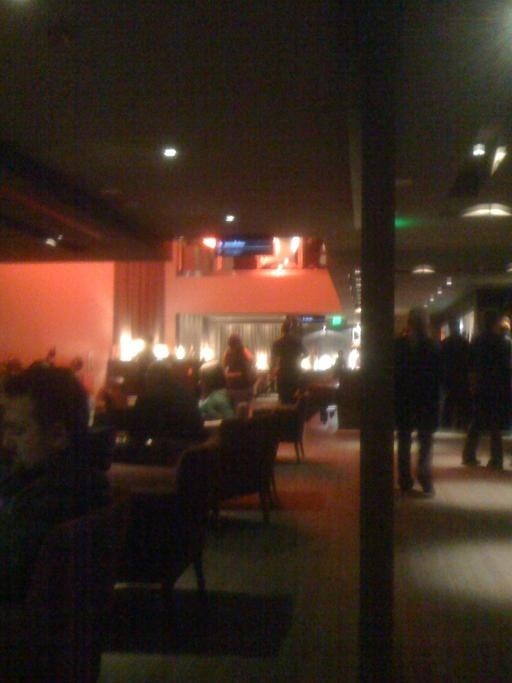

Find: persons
<box><xmin>393</xmin><ymin>310</ymin><xmax>512</xmax><ymax>494</ymax></box>
<box><xmin>270</xmin><ymin>318</ymin><xmax>307</xmax><ymax>403</ymax></box>
<box><xmin>0</xmin><ymin>354</ymin><xmax>236</xmax><ymax>683</ymax></box>
<box><xmin>222</xmin><ymin>334</ymin><xmax>258</xmax><ymax>402</ymax></box>
